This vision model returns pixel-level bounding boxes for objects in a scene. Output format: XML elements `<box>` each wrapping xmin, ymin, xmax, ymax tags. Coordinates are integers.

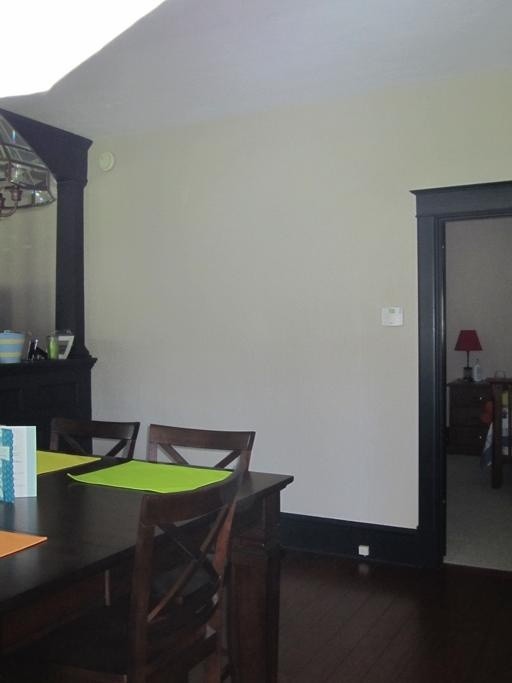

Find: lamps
<box><xmin>452</xmin><ymin>329</ymin><xmax>482</xmax><ymax>383</ymax></box>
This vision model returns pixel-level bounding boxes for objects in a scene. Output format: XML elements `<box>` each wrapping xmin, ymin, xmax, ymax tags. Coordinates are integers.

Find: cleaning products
<box><xmin>473</xmin><ymin>359</ymin><xmax>482</xmax><ymax>381</ymax></box>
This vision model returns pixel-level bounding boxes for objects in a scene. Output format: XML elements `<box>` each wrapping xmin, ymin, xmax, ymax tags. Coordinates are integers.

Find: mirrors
<box><xmin>0</xmin><ymin>111</ymin><xmax>58</xmax><ymax>359</ymax></box>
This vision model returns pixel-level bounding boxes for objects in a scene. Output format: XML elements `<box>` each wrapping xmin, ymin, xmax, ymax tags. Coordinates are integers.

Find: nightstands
<box><xmin>445</xmin><ymin>379</ymin><xmax>492</xmax><ymax>456</ymax></box>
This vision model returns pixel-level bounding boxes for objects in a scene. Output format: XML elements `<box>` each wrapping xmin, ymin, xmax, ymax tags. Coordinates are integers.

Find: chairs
<box><xmin>140</xmin><ymin>424</ymin><xmax>255</xmax><ymax>644</ymax></box>
<box><xmin>48</xmin><ymin>420</ymin><xmax>140</xmax><ymax>610</ymax></box>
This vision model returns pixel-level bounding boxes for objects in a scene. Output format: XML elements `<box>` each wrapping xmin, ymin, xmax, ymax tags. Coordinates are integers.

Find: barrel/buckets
<box><xmin>0</xmin><ymin>330</ymin><xmax>26</xmax><ymax>363</ymax></box>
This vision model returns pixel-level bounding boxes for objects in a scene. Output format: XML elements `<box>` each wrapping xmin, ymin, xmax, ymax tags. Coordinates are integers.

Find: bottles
<box><xmin>46</xmin><ymin>335</ymin><xmax>59</xmax><ymax>360</ymax></box>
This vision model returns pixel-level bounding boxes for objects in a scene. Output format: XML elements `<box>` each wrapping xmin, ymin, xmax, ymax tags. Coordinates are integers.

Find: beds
<box><xmin>491</xmin><ymin>376</ymin><xmax>512</xmax><ymax>488</ymax></box>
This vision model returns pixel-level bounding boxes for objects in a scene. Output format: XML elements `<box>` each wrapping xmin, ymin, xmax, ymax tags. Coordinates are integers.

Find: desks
<box><xmin>0</xmin><ymin>448</ymin><xmax>294</xmax><ymax>682</ymax></box>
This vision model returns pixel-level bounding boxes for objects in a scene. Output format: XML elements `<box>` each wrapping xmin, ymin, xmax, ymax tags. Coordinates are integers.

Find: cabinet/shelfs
<box><xmin>0</xmin><ymin>357</ymin><xmax>99</xmax><ymax>456</ymax></box>
